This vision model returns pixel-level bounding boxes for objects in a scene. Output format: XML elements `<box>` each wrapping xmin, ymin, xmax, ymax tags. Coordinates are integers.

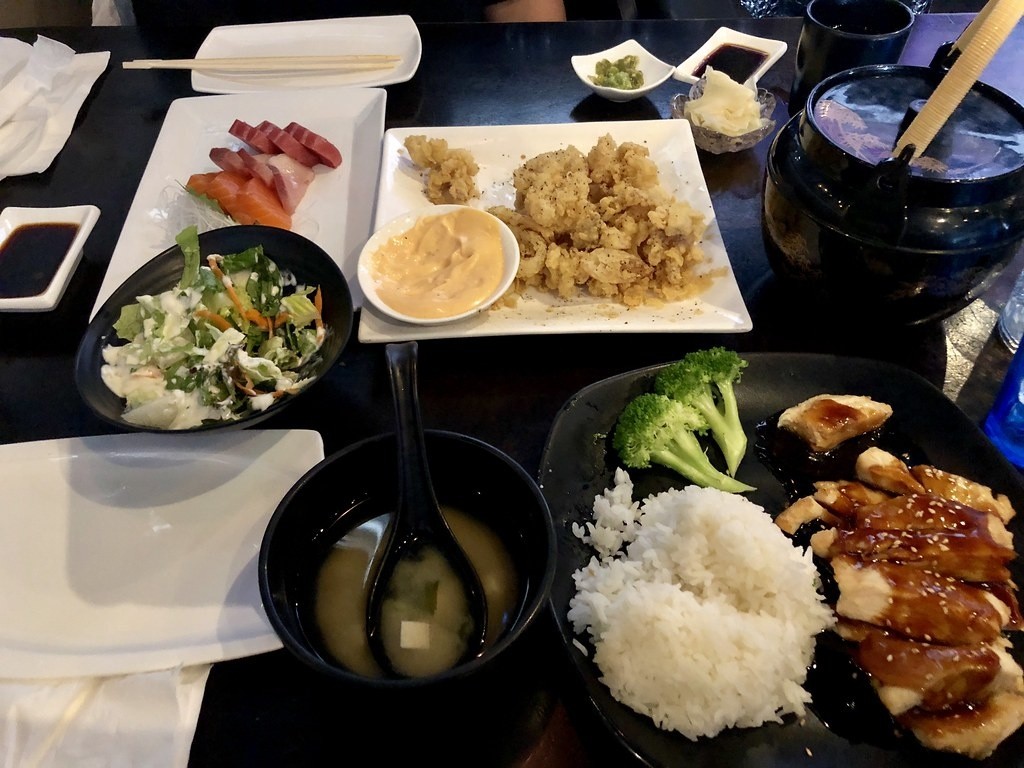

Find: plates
<box><xmin>571</xmin><ymin>40</ymin><xmax>674</xmax><ymax>103</ymax></box>
<box><xmin>360</xmin><ymin>119</ymin><xmax>754</xmax><ymax>344</ymax></box>
<box><xmin>89</xmin><ymin>88</ymin><xmax>387</xmax><ymax>325</ymax></box>
<box><xmin>672</xmin><ymin>25</ymin><xmax>788</xmax><ymax>86</ymax></box>
<box><xmin>538</xmin><ymin>351</ymin><xmax>1022</xmax><ymax>765</ymax></box>
<box><xmin>192</xmin><ymin>15</ymin><xmax>422</xmax><ymax>94</ymax></box>
<box><xmin>1</xmin><ymin>205</ymin><xmax>101</xmax><ymax>310</ymax></box>
<box><xmin>1</xmin><ymin>428</ymin><xmax>324</xmax><ymax>680</ymax></box>
<box><xmin>357</xmin><ymin>204</ymin><xmax>520</xmax><ymax>324</ymax></box>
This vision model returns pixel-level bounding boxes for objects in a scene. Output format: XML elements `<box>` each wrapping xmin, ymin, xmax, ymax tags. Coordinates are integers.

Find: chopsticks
<box><xmin>122</xmin><ymin>55</ymin><xmax>401</xmax><ymax>70</ymax></box>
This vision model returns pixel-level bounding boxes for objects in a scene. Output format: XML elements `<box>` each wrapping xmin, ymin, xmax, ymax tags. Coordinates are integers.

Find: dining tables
<box><xmin>0</xmin><ymin>12</ymin><xmax>1024</xmax><ymax>767</ymax></box>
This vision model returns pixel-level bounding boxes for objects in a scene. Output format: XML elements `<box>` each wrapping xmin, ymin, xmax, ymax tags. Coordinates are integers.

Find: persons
<box><xmin>0</xmin><ymin>0</ymin><xmax>568</xmax><ymax>28</ymax></box>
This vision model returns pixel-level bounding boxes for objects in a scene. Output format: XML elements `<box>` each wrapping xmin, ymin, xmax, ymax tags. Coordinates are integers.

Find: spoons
<box><xmin>366</xmin><ymin>340</ymin><xmax>487</xmax><ymax>680</ymax></box>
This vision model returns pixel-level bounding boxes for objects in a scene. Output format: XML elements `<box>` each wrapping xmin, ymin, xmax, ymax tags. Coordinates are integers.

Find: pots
<box><xmin>763</xmin><ymin>40</ymin><xmax>1023</xmax><ymax>324</ymax></box>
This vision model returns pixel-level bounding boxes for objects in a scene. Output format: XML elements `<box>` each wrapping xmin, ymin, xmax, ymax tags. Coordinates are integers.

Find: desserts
<box><xmin>682</xmin><ymin>65</ymin><xmax>767</xmax><ymax>137</ymax></box>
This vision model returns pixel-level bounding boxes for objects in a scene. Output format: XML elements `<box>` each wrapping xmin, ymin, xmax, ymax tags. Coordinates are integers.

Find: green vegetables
<box><xmin>113</xmin><ymin>224</ymin><xmax>324</xmax><ymax>423</ymax></box>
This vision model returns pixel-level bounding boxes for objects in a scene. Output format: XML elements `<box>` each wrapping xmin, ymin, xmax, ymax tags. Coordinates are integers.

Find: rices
<box><xmin>567</xmin><ymin>467</ymin><xmax>837</xmax><ymax>742</ymax></box>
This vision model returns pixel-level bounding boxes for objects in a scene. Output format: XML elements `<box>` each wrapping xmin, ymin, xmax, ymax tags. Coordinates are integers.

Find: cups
<box><xmin>997</xmin><ymin>268</ymin><xmax>1023</xmax><ymax>352</ymax></box>
<box><xmin>789</xmin><ymin>1</ymin><xmax>914</xmax><ymax>101</ymax></box>
<box><xmin>983</xmin><ymin>335</ymin><xmax>1023</xmax><ymax>468</ymax></box>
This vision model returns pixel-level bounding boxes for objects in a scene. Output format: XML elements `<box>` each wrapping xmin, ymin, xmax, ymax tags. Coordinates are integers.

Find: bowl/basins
<box><xmin>73</xmin><ymin>226</ymin><xmax>354</xmax><ymax>437</ymax></box>
<box><xmin>258</xmin><ymin>431</ymin><xmax>557</xmax><ymax>687</ymax></box>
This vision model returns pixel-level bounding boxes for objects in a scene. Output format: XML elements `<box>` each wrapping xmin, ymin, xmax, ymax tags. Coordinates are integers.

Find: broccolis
<box><xmin>613</xmin><ymin>346</ymin><xmax>758</xmax><ymax>494</ymax></box>
